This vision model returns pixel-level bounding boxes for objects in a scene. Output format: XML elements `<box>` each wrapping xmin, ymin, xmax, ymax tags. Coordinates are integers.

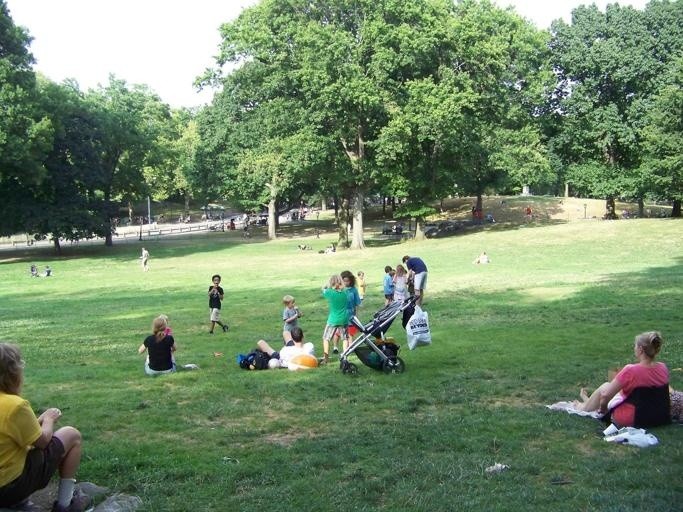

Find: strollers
<box><xmin>338</xmin><ymin>294</ymin><xmax>418</xmax><ymax>375</ymax></box>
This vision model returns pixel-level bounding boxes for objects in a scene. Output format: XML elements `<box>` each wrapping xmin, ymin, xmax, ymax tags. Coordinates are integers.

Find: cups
<box><xmin>602</xmin><ymin>423</ymin><xmax>618</xmax><ymax>436</ymax></box>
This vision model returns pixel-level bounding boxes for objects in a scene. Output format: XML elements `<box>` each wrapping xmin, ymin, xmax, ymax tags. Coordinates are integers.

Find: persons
<box><xmin>333</xmin><ymin>270</ymin><xmax>361</xmax><ymax>353</ymax></box>
<box><xmin>471</xmin><ymin>201</ymin><xmax>532</xmax><ymax>226</ymax></box>
<box><xmin>139</xmin><ymin>247</ymin><xmax>150</xmax><ymax>271</ymax></box>
<box><xmin>112</xmin><ymin>208</ymin><xmax>335</xmax><ymax>254</ymax></box>
<box><xmin>357</xmin><ymin>247</ymin><xmax>428</xmax><ymax>305</ymax></box>
<box><xmin>319</xmin><ymin>275</ymin><xmax>350</xmax><ymax>365</ymax></box>
<box><xmin>208</xmin><ymin>275</ymin><xmax>229</xmax><ymax>333</ymax></box>
<box><xmin>256</xmin><ymin>327</ymin><xmax>314</xmax><ymax>368</ymax></box>
<box><xmin>476</xmin><ymin>252</ymin><xmax>489</xmax><ymax>264</ymax></box>
<box><xmin>160</xmin><ymin>313</ymin><xmax>172</xmax><ymax>336</ymax></box>
<box><xmin>0</xmin><ymin>344</ymin><xmax>96</xmax><ymax>512</ymax></box>
<box><xmin>31</xmin><ymin>266</ymin><xmax>51</xmax><ymax>276</ymax></box>
<box><xmin>282</xmin><ymin>295</ymin><xmax>304</xmax><ymax>331</ymax></box>
<box><xmin>138</xmin><ymin>318</ymin><xmax>177</xmax><ymax>376</ymax></box>
<box><xmin>603</xmin><ymin>204</ymin><xmax>667</xmax><ymax>220</ymax></box>
<box><xmin>573</xmin><ymin>331</ymin><xmax>671</xmax><ymax>428</ymax></box>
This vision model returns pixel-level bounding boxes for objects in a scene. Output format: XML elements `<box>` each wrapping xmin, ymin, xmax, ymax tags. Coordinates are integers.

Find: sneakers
<box><xmin>51</xmin><ymin>489</ymin><xmax>91</xmax><ymax>512</ymax></box>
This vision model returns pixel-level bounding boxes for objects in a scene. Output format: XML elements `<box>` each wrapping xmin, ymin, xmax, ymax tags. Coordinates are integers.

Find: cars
<box><xmin>381</xmin><ymin>220</ymin><xmax>402</xmax><ymax>234</ymax></box>
